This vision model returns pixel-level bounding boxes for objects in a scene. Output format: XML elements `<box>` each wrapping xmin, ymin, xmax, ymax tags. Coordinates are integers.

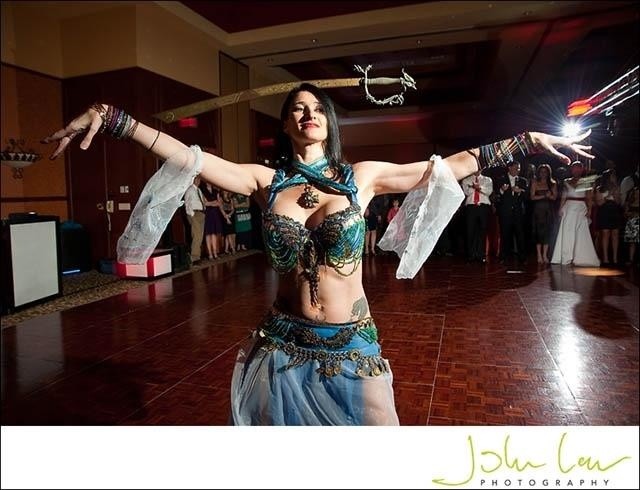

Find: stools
<box><xmin>113</xmin><ymin>246</ymin><xmax>174</xmax><ymax>280</ymax></box>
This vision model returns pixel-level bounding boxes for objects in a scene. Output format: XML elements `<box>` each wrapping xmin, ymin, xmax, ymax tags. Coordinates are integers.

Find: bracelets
<box><xmin>467</xmin><ymin>131</ymin><xmax>540</xmax><ymax>175</ymax></box>
<box><xmin>90</xmin><ymin>100</ymin><xmax>164</xmax><ymax>155</ymax></box>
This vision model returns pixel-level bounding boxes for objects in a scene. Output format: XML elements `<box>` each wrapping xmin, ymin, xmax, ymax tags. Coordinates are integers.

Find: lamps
<box><xmin>2</xmin><ymin>139</ymin><xmax>40</xmax><ymax>181</ymax></box>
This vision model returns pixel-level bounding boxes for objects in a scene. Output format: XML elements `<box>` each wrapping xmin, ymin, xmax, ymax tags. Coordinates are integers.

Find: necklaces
<box><xmin>296</xmin><ymin>181</ymin><xmax>318</xmax><ymax>208</ymax></box>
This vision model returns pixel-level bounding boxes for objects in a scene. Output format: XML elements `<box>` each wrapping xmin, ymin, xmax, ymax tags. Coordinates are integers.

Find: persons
<box><xmin>37</xmin><ymin>79</ymin><xmax>593</xmax><ymax>425</ymax></box>
<box><xmin>233</xmin><ymin>192</ymin><xmax>253</xmax><ymax>251</ymax></box>
<box><xmin>215</xmin><ymin>190</ymin><xmax>236</xmax><ymax>258</ymax></box>
<box><xmin>182</xmin><ymin>170</ymin><xmax>206</xmax><ymax>267</ymax></box>
<box><xmin>363</xmin><ymin>153</ymin><xmax>640</xmax><ymax>269</ymax></box>
<box><xmin>201</xmin><ymin>177</ymin><xmax>222</xmax><ymax>263</ymax></box>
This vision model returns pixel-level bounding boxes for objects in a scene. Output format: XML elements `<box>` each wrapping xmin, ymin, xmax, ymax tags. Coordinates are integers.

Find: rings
<box><xmin>63</xmin><ymin>126</ymin><xmax>76</xmax><ymax>135</ymax></box>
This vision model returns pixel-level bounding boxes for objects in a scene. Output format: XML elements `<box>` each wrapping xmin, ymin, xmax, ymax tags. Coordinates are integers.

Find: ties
<box><xmin>474</xmin><ymin>176</ymin><xmax>480</xmax><ymax>207</ymax></box>
<box><xmin>198</xmin><ymin>190</ymin><xmax>204</xmax><ymax>212</ymax></box>
